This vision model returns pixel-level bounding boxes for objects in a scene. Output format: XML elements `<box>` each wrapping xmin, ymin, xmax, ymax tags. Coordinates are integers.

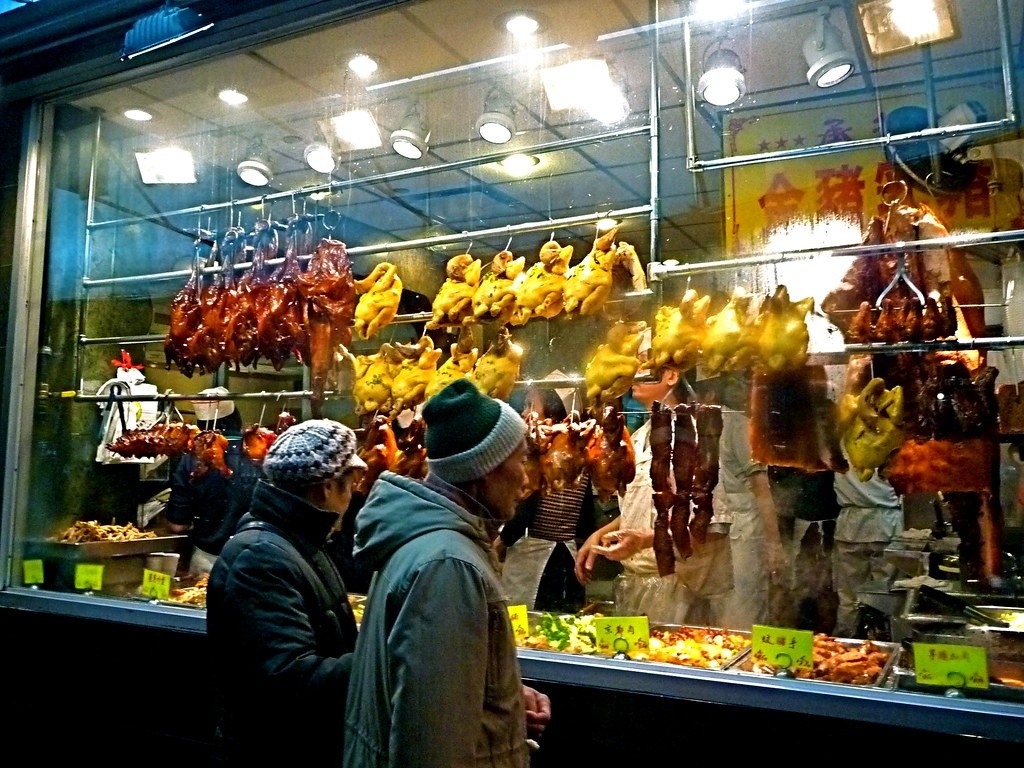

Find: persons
<box><xmin>571</xmin><ymin>361</ymin><xmax>733</xmax><ymax>625</ymax></box>
<box><xmin>686</xmin><ymin>369</ymin><xmax>790</xmax><ymax>629</ymax></box>
<box><xmin>773</xmin><ymin>463</ymin><xmax>841</xmax><ymax>635</ymax></box>
<box><xmin>829</xmin><ymin>447</ymin><xmax>904</xmax><ymax>638</ymax></box>
<box><xmin>203</xmin><ymin>420</ymin><xmax>368</xmax><ymax>768</ymax></box>
<box><xmin>492</xmin><ymin>368</ymin><xmax>597</xmax><ymax>615</ymax></box>
<box><xmin>339</xmin><ymin>376</ymin><xmax>552</xmax><ymax>768</ymax></box>
<box><xmin>163</xmin><ymin>383</ymin><xmax>429</xmax><ymax>596</ymax></box>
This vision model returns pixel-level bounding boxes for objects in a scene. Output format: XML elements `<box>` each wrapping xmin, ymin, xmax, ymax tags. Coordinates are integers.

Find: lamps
<box><xmin>390</xmin><ymin>97</ymin><xmax>432</xmax><ymax>160</ymax></box>
<box><xmin>698</xmin><ymin>28</ymin><xmax>747</xmax><ymax>106</ymax></box>
<box><xmin>802</xmin><ymin>6</ymin><xmax>857</xmax><ymax>89</ymax></box>
<box><xmin>855</xmin><ymin>0</ymin><xmax>961</xmax><ymax>59</ymax></box>
<box><xmin>476</xmin><ymin>87</ymin><xmax>516</xmax><ymax>143</ymax></box>
<box><xmin>235</xmin><ymin>135</ymin><xmax>272</xmax><ymax>187</ymax></box>
<box><xmin>304</xmin><ymin>133</ymin><xmax>343</xmax><ymax>174</ymax></box>
<box><xmin>134</xmin><ymin>147</ymin><xmax>198</xmax><ymax>185</ymax></box>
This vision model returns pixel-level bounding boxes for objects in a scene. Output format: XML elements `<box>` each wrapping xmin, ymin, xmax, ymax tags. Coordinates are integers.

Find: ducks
<box><xmin>164</xmin><ymin>213</ymin><xmax>354</xmax><ymax>405</ymax></box>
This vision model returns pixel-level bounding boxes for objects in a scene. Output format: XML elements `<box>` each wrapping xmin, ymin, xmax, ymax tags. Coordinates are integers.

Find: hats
<box><xmin>192</xmin><ymin>386</ymin><xmax>234</xmax><ymax>421</ymax></box>
<box><xmin>422</xmin><ymin>378</ymin><xmax>529</xmax><ymax>483</ymax></box>
<box><xmin>545</xmin><ymin>369</ymin><xmax>583</xmax><ymax>420</ymax></box>
<box><xmin>263</xmin><ymin>419</ymin><xmax>369</xmax><ymax>487</ymax></box>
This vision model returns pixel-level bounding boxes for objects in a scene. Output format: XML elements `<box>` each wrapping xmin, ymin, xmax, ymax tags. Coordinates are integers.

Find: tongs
<box><xmin>919</xmin><ymin>583</ymin><xmax>1011</xmax><ymax>628</ymax></box>
